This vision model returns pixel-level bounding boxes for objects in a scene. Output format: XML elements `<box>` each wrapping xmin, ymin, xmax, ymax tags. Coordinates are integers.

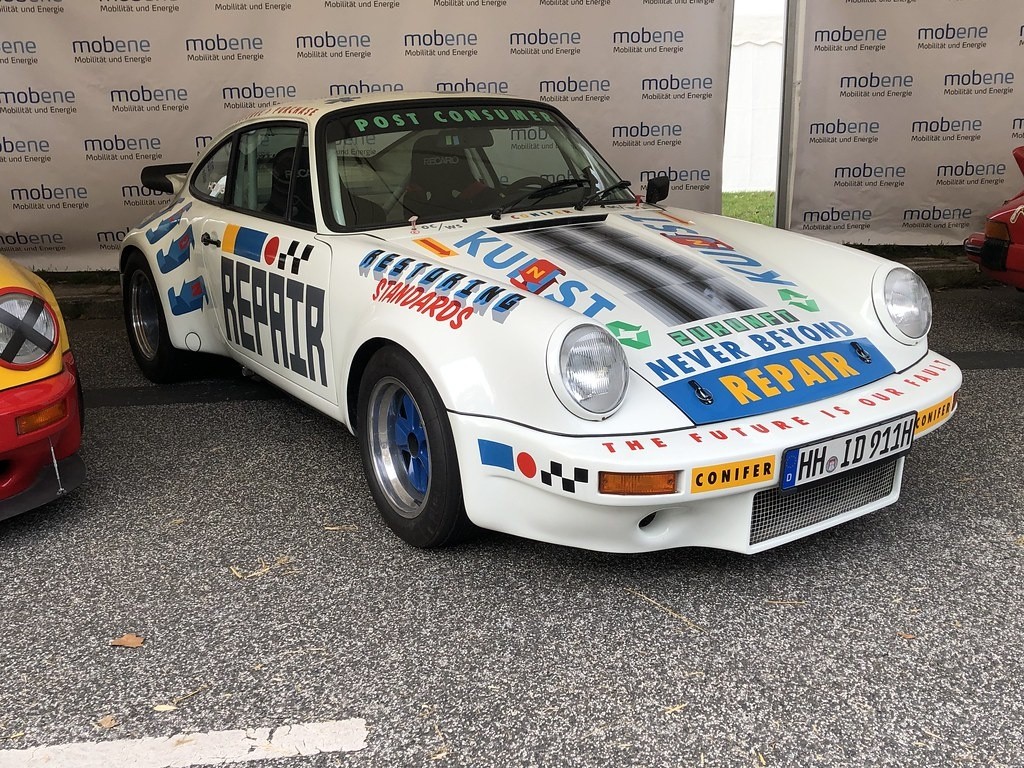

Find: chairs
<box><xmin>404</xmin><ymin>135</ymin><xmax>476</xmax><ymax>227</ymax></box>
<box><xmin>264</xmin><ymin>146</ymin><xmax>388</xmax><ymax>232</ymax></box>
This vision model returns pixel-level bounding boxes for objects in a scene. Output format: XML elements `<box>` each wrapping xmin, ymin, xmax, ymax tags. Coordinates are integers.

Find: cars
<box><xmin>0</xmin><ymin>251</ymin><xmax>89</xmax><ymax>522</ymax></box>
<box><xmin>963</xmin><ymin>145</ymin><xmax>1024</xmax><ymax>291</ymax></box>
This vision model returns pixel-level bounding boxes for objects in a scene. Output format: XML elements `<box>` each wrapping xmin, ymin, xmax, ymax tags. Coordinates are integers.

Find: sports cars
<box><xmin>118</xmin><ymin>90</ymin><xmax>966</xmax><ymax>556</ymax></box>
